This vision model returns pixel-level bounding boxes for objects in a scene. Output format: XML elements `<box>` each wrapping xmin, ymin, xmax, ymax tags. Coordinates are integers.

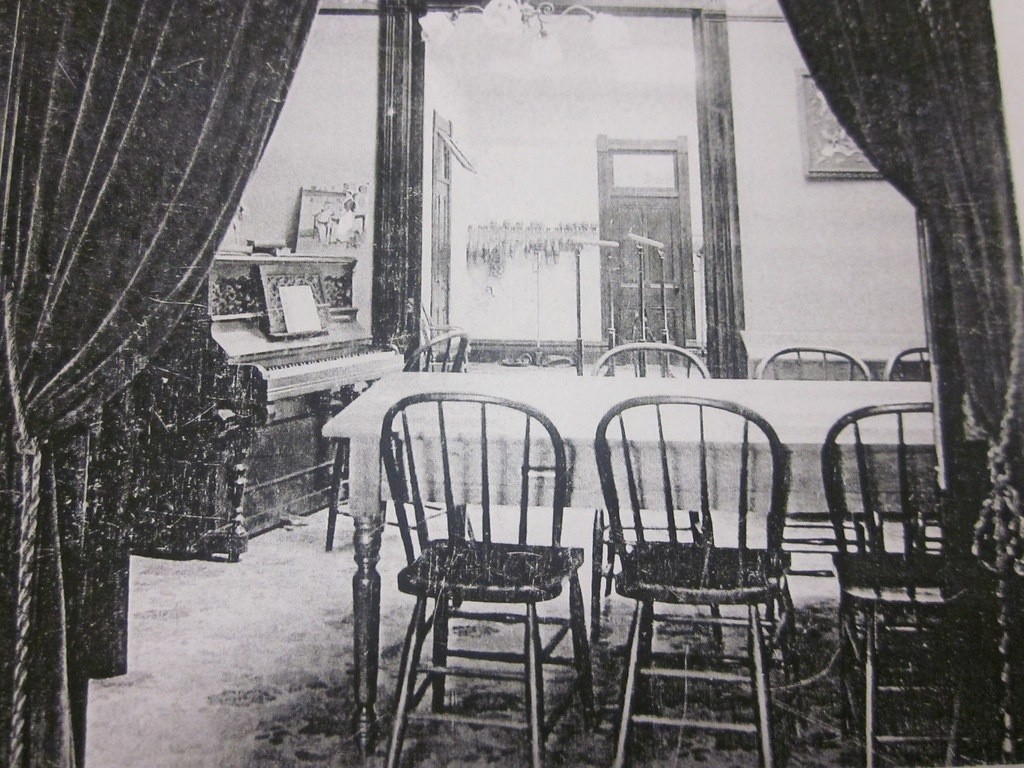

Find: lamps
<box><xmin>419</xmin><ymin>0</ymin><xmax>621</xmax><ymax>48</ymax></box>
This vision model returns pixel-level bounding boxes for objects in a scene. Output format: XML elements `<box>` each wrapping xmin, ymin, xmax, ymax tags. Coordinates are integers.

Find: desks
<box><xmin>321</xmin><ymin>371</ymin><xmax>939</xmax><ymax>762</ymax></box>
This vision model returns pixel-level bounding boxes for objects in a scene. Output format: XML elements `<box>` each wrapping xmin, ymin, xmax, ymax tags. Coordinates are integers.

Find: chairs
<box><xmin>325</xmin><ymin>332</ymin><xmax>982</xmax><ymax>767</ymax></box>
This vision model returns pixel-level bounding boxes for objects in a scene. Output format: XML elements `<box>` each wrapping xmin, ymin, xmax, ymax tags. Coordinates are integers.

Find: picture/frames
<box><xmin>794</xmin><ymin>66</ymin><xmax>884</xmax><ymax>179</ymax></box>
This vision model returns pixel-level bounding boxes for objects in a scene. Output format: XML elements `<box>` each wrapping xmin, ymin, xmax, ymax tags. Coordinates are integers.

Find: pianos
<box><xmin>123</xmin><ymin>247</ymin><xmax>408</xmax><ymax>564</ymax></box>
